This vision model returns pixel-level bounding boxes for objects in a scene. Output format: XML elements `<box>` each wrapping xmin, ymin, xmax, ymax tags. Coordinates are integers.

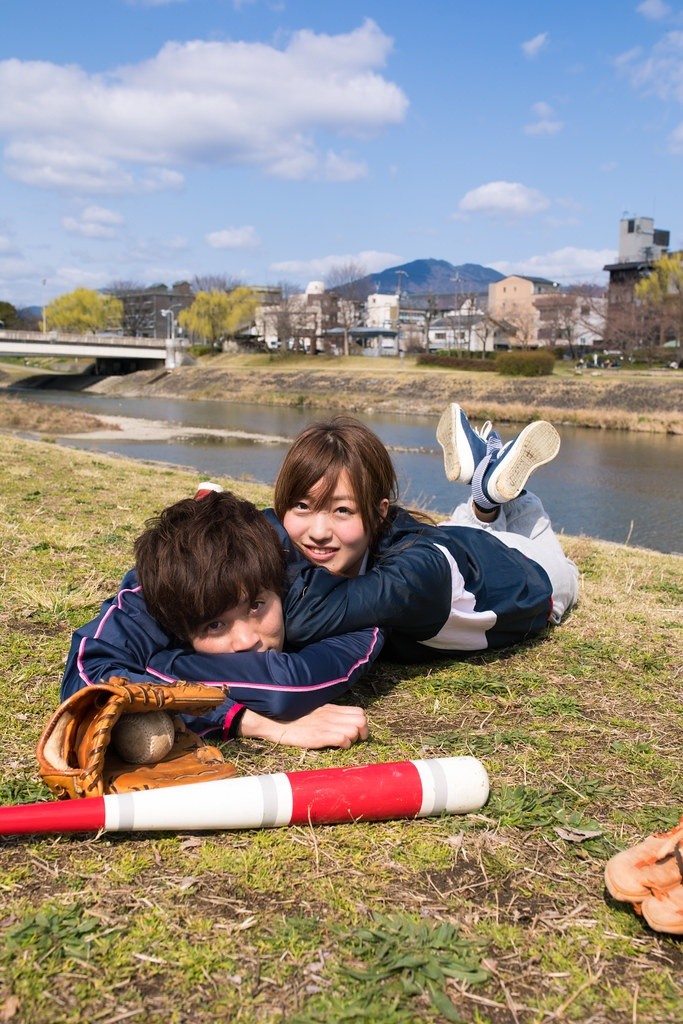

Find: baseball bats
<box><xmin>1</xmin><ymin>756</ymin><xmax>492</xmax><ymax>836</ymax></box>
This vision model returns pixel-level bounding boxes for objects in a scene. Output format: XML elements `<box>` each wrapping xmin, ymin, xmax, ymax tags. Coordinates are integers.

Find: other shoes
<box><xmin>192</xmin><ymin>482</ymin><xmax>225</xmax><ymax>501</ymax></box>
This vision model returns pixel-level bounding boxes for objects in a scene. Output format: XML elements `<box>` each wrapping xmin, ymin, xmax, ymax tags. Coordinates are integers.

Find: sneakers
<box><xmin>436</xmin><ymin>402</ymin><xmax>499</xmax><ymax>484</ymax></box>
<box><xmin>472</xmin><ymin>420</ymin><xmax>561</xmax><ymax>508</ymax></box>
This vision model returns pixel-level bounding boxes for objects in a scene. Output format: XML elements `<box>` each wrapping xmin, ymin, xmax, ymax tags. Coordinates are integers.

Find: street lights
<box><xmin>160</xmin><ymin>303</ymin><xmax>182</xmax><ymax>339</ymax></box>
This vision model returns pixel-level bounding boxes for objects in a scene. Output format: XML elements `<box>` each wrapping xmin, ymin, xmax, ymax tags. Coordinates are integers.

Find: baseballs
<box><xmin>109</xmin><ymin>708</ymin><xmax>176</xmax><ymax>764</ymax></box>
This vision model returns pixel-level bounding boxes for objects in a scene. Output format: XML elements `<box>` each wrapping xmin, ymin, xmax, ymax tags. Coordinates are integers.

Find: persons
<box><xmin>59</xmin><ymin>482</ymin><xmax>471</xmax><ymax>750</ymax></box>
<box><xmin>257</xmin><ymin>403</ymin><xmax>578</xmax><ymax>665</ymax></box>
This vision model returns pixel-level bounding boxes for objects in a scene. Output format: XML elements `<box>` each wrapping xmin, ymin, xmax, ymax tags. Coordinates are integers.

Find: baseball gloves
<box><xmin>603</xmin><ymin>821</ymin><xmax>683</xmax><ymax>934</ymax></box>
<box><xmin>35</xmin><ymin>674</ymin><xmax>239</xmax><ymax>801</ymax></box>
